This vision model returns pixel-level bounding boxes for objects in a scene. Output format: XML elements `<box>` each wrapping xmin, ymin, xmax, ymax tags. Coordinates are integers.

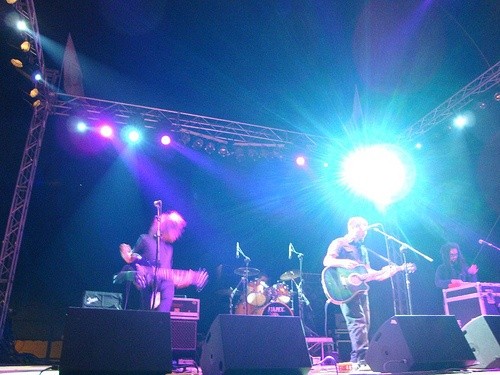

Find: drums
<box><xmin>268</xmin><ymin>283</ymin><xmax>291</xmax><ymax>303</ymax></box>
<box><xmin>258</xmin><ymin>302</ymin><xmax>293</xmax><ymax>316</ymax></box>
<box><xmin>248</xmin><ymin>280</ymin><xmax>267</xmax><ymax>306</ymax></box>
<box><xmin>236</xmin><ymin>303</ymin><xmax>255</xmax><ymax>314</ymax></box>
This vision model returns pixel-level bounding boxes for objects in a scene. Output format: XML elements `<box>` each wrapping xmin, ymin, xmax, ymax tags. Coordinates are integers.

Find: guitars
<box><xmin>321</xmin><ymin>262</ymin><xmax>417</xmax><ymax>305</ymax></box>
<box><xmin>121</xmin><ymin>259</ymin><xmax>209</xmax><ymax>292</ymax></box>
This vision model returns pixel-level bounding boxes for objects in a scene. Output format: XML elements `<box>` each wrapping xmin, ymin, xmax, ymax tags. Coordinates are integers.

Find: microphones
<box><xmin>236</xmin><ymin>241</ymin><xmax>240</xmax><ymax>258</ymax></box>
<box><xmin>479</xmin><ymin>240</ymin><xmax>493</xmax><ymax>246</ymax></box>
<box><xmin>288</xmin><ymin>243</ymin><xmax>293</xmax><ymax>259</ymax></box>
<box><xmin>367</xmin><ymin>224</ymin><xmax>379</xmax><ymax>230</ymax></box>
<box><xmin>154</xmin><ymin>200</ymin><xmax>161</xmax><ymax>205</ymax></box>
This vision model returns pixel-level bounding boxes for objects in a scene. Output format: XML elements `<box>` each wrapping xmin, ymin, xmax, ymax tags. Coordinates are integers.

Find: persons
<box><xmin>235</xmin><ymin>273</ymin><xmax>279</xmax><ymax>315</ymax></box>
<box><xmin>435</xmin><ymin>241</ymin><xmax>478</xmax><ymax>288</ymax></box>
<box><xmin>322</xmin><ymin>215</ymin><xmax>400</xmax><ymax>361</ymax></box>
<box><xmin>118</xmin><ymin>210</ymin><xmax>186</xmax><ymax>310</ymax></box>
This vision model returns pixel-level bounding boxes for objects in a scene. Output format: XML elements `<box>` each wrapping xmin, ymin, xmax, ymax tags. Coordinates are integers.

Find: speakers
<box><xmin>460</xmin><ymin>314</ymin><xmax>500</xmax><ymax>367</ymax></box>
<box><xmin>336</xmin><ymin>332</ymin><xmax>354</xmax><ymax>362</ymax></box>
<box><xmin>61</xmin><ymin>306</ymin><xmax>173</xmax><ymax>375</ymax></box>
<box><xmin>364</xmin><ymin>316</ymin><xmax>476</xmax><ymax>371</ymax></box>
<box><xmin>170</xmin><ymin>319</ymin><xmax>198</xmax><ymax>350</ymax></box>
<box><xmin>199</xmin><ymin>314</ymin><xmax>312</xmax><ymax>375</ymax></box>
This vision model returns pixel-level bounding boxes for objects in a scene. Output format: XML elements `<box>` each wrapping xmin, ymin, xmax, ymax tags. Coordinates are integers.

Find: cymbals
<box><xmin>280</xmin><ymin>270</ymin><xmax>303</xmax><ymax>280</ymax></box>
<box><xmin>215</xmin><ymin>290</ymin><xmax>240</xmax><ymax>296</ymax></box>
<box><xmin>234</xmin><ymin>267</ymin><xmax>260</xmax><ymax>276</ymax></box>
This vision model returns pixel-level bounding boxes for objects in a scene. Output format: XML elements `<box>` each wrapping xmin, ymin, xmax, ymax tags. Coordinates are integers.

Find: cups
<box><xmin>312</xmin><ymin>357</ymin><xmax>320</xmax><ymax>369</ymax></box>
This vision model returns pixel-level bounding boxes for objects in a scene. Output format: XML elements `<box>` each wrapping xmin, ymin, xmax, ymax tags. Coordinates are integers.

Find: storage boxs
<box><xmin>170</xmin><ymin>298</ymin><xmax>200</xmax><ymax>320</ymax></box>
<box><xmin>334</xmin><ymin>328</ymin><xmax>352</xmax><ymax>362</ymax></box>
<box><xmin>442</xmin><ymin>282</ymin><xmax>500</xmax><ymax>328</ymax></box>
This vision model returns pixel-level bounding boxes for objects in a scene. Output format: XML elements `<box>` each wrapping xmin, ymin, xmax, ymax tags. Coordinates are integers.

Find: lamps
<box><xmin>178</xmin><ymin>132</ymin><xmax>288</xmax><ymax>163</ymax></box>
<box><xmin>0</xmin><ymin>0</ymin><xmax>41</xmax><ymax>107</ymax></box>
